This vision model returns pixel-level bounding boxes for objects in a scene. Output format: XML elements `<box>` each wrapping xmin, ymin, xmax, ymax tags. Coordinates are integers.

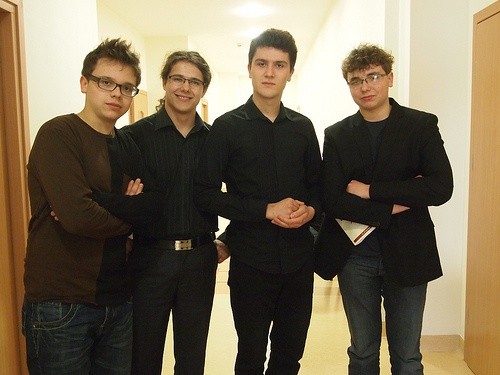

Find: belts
<box><xmin>149</xmin><ymin>232</ymin><xmax>216</xmax><ymax>251</ymax></box>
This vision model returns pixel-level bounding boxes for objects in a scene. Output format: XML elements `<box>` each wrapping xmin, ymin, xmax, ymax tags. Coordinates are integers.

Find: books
<box><xmin>336</xmin><ymin>219</ymin><xmax>376</xmax><ymax>246</ymax></box>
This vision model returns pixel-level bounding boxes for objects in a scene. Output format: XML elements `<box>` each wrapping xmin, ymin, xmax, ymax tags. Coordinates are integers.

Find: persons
<box><xmin>194</xmin><ymin>28</ymin><xmax>323</xmax><ymax>375</ymax></box>
<box><xmin>317</xmin><ymin>44</ymin><xmax>454</xmax><ymax>375</ymax></box>
<box><xmin>121</xmin><ymin>51</ymin><xmax>230</xmax><ymax>375</ymax></box>
<box><xmin>22</xmin><ymin>38</ymin><xmax>143</xmax><ymax>375</ymax></box>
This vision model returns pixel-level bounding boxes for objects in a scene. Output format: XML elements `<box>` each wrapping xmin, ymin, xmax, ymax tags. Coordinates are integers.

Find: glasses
<box><xmin>86</xmin><ymin>73</ymin><xmax>139</xmax><ymax>98</ymax></box>
<box><xmin>165</xmin><ymin>72</ymin><xmax>207</xmax><ymax>89</ymax></box>
<box><xmin>347</xmin><ymin>71</ymin><xmax>387</xmax><ymax>87</ymax></box>
